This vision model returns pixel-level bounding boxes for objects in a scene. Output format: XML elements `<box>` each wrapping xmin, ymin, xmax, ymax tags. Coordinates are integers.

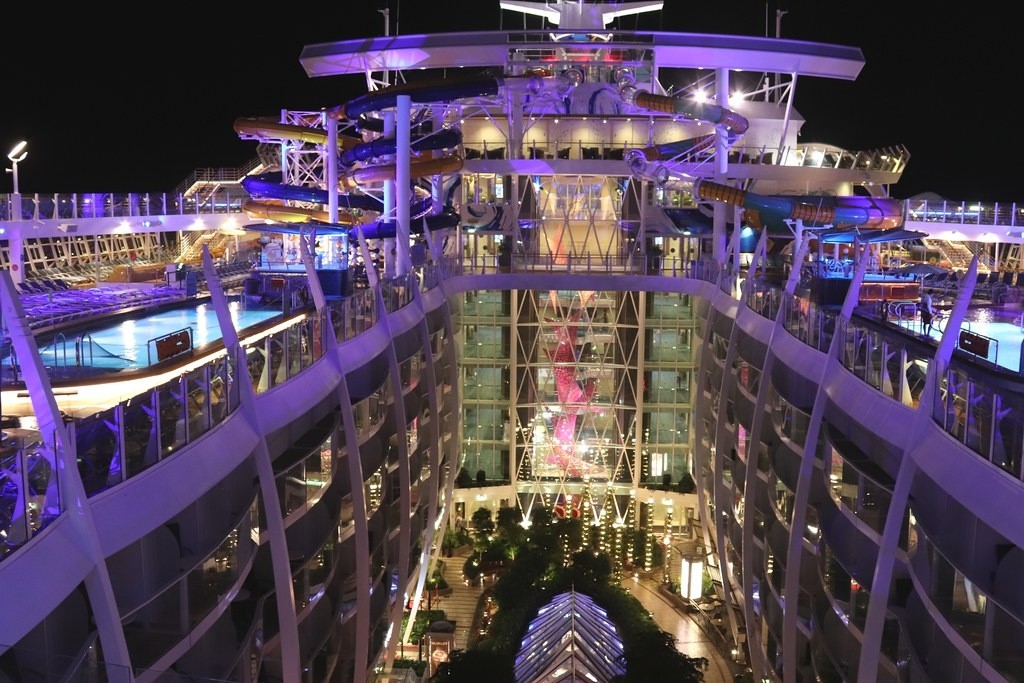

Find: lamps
<box><xmin>673</xmin><ymin>543</ymin><xmax>708</xmax><ymax>603</ymax></box>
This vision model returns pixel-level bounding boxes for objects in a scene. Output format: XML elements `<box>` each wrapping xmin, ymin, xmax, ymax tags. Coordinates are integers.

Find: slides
<box><xmin>232</xmin><ymin>69</ymin><xmax>500</xmax><ymax>241</ymax></box>
<box><xmin>615</xmin><ymin>69</ymin><xmax>903</xmax><ymax>228</ymax></box>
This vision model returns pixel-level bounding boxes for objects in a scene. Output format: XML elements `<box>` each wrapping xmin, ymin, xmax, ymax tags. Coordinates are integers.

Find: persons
<box><xmin>923</xmin><ymin>288</ymin><xmax>936</xmax><ymax>337</ymax></box>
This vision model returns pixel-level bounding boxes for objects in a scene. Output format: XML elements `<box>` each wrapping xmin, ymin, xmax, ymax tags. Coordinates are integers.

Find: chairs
<box><xmin>738</xmin><ymin>252</ymin><xmax>1023</xmax><ymax>324</ymax></box>
<box><xmin>0</xmin><ymin>254</ymin><xmax>255</xmax><ymax>342</ymax></box>
